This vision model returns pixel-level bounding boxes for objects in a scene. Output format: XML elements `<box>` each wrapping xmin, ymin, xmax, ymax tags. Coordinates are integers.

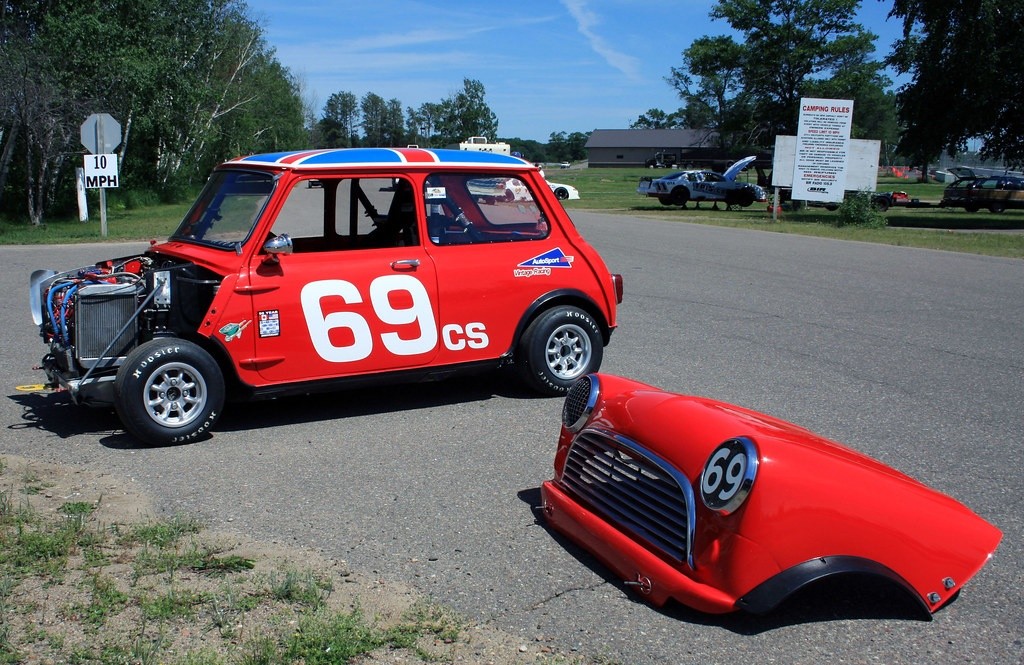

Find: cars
<box><xmin>561</xmin><ymin>162</ymin><xmax>570</xmax><ymax>168</ymax></box>
<box><xmin>15</xmin><ymin>147</ymin><xmax>626</xmax><ymax>446</ymax></box>
<box><xmin>823</xmin><ymin>191</ymin><xmax>891</xmax><ymax>212</ymax></box>
<box><xmin>943</xmin><ymin>167</ymin><xmax>1024</xmax><ymax>213</ymax></box>
<box><xmin>466</xmin><ymin>179</ymin><xmax>506</xmax><ymax>205</ymax></box>
<box><xmin>637</xmin><ymin>156</ymin><xmax>767</xmax><ymax>207</ymax></box>
<box><xmin>497</xmin><ymin>178</ymin><xmax>580</xmax><ymax>202</ymax></box>
<box><xmin>644</xmin><ymin>158</ymin><xmax>666</xmax><ymax>168</ymax></box>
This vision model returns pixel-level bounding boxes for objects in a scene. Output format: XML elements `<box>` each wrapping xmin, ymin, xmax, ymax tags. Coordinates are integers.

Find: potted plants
<box><xmin>767</xmin><ymin>194</ymin><xmax>782</xmax><ymax>214</ymax></box>
